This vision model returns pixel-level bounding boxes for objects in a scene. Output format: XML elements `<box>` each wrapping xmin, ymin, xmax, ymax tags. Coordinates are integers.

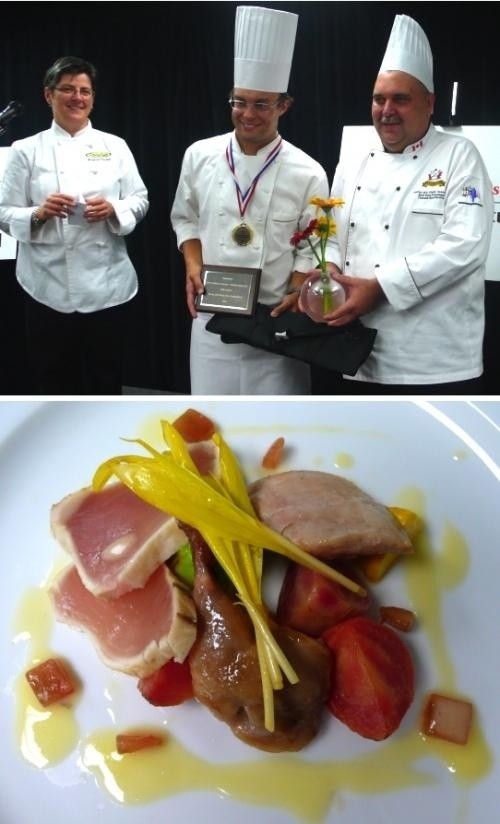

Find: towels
<box><xmin>205</xmin><ymin>300</ymin><xmax>380</xmax><ymax>377</ymax></box>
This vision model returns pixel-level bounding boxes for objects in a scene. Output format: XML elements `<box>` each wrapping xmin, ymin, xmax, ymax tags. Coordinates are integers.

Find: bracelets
<box><xmin>287</xmin><ymin>287</ymin><xmax>300</xmax><ymax>295</ymax></box>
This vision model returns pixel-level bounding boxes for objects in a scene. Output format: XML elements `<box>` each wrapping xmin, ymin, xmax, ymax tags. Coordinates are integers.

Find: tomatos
<box><xmin>279</xmin><ymin>556</ymin><xmax>413</xmax><ymax>741</ymax></box>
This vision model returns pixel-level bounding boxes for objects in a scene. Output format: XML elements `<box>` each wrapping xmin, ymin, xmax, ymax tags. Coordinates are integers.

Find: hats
<box><xmin>231</xmin><ymin>4</ymin><xmax>300</xmax><ymax>95</ymax></box>
<box><xmin>375</xmin><ymin>13</ymin><xmax>437</xmax><ymax>94</ymax></box>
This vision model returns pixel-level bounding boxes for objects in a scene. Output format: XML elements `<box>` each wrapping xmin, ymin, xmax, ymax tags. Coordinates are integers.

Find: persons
<box><xmin>0</xmin><ymin>56</ymin><xmax>150</xmax><ymax>396</ymax></box>
<box><xmin>169</xmin><ymin>89</ymin><xmax>329</xmax><ymax>396</ymax></box>
<box><xmin>298</xmin><ymin>71</ymin><xmax>495</xmax><ymax>395</ymax></box>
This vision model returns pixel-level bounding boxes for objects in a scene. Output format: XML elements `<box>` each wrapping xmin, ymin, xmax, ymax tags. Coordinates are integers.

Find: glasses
<box><xmin>228</xmin><ymin>95</ymin><xmax>284</xmax><ymax>112</ymax></box>
<box><xmin>51</xmin><ymin>85</ymin><xmax>92</xmax><ymax>96</ymax></box>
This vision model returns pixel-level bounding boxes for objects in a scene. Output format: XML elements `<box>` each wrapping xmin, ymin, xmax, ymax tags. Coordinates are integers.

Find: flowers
<box><xmin>287</xmin><ymin>194</ymin><xmax>345</xmax><ymax>316</ymax></box>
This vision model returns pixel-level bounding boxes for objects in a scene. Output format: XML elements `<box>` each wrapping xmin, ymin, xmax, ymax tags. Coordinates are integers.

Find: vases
<box><xmin>299</xmin><ymin>270</ymin><xmax>346</xmax><ymax>322</ymax></box>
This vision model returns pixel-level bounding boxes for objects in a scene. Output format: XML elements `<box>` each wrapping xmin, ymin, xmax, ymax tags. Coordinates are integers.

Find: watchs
<box><xmin>32</xmin><ymin>209</ymin><xmax>46</xmax><ymax>227</ymax></box>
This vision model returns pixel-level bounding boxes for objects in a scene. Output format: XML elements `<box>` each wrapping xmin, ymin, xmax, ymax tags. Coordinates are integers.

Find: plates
<box><xmin>2</xmin><ymin>396</ymin><xmax>500</xmax><ymax>820</ymax></box>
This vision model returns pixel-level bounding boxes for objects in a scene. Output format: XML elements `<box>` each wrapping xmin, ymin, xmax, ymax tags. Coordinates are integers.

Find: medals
<box><xmin>231</xmin><ymin>223</ymin><xmax>253</xmax><ymax>246</ymax></box>
